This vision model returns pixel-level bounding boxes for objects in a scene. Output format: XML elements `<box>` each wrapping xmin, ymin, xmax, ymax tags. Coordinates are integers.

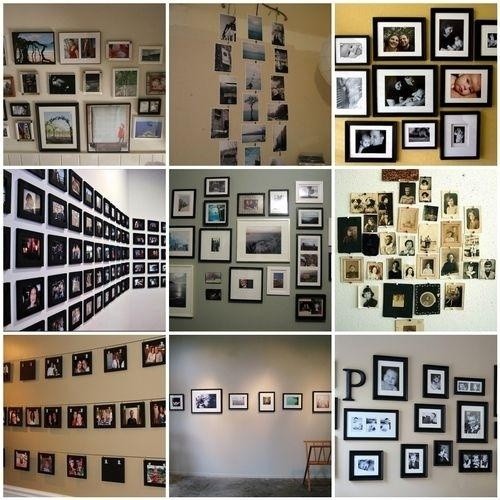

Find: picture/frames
<box><xmin>170</xmin><ymin>387</ymin><xmax>332</xmax><ymax>416</ymax></box>
<box><xmin>332</xmin><ymin>5</ymin><xmax>497</xmax><ymax>162</ymax></box>
<box><xmin>3</xmin><ymin>335</ymin><xmax>167</xmax><ymax>488</ymax></box>
<box><xmin>333</xmin><ymin>353</ymin><xmax>497</xmax><ymax>483</ymax></box>
<box><xmin>3</xmin><ymin>28</ymin><xmax>164</xmax><ymax>153</ymax></box>
<box><xmin>3</xmin><ymin>168</ymin><xmax>168</xmax><ymax>333</ymax></box>
<box><xmin>168</xmin><ymin>174</ymin><xmax>332</xmax><ymax>322</ymax></box>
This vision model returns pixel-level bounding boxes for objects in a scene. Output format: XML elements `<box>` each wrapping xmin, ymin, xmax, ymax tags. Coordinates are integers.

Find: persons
<box><xmin>343</xmin><ymin>180</ymin><xmax>495</xmax><ymax>308</ymax></box>
<box><xmin>340</xmin><ymin>25</ymin><xmax>496</xmax><ymax>153</ymax></box>
<box><xmin>68</xmin><ymin>38</ymin><xmax>79</xmax><ymax>59</ymax></box>
<box><xmin>117</xmin><ymin>124</ymin><xmax>125</xmax><ymax>144</ymax></box>
<box><xmin>24</xmin><ymin>173</ymin><xmax>166</xmax><ymax>331</ymax></box>
<box><xmin>208</xmin><ymin>182</ymin><xmax>321</xmax><ymax>314</ymax></box>
<box><xmin>352</xmin><ymin>366</ymin><xmax>489</xmax><ymax>471</ymax></box>
<box><xmin>25</xmin><ymin>76</ymin><xmax>33</xmax><ymax>91</ymax></box>
<box><xmin>151</xmin><ymin>77</ymin><xmax>165</xmax><ymax>90</ymax></box>
<box><xmin>109</xmin><ymin>44</ymin><xmax>128</xmax><ymax>58</ymax></box>
<box><xmin>3</xmin><ymin>342</ymin><xmax>166</xmax><ymax>483</ymax></box>
<box><xmin>52</xmin><ymin>78</ymin><xmax>67</xmax><ymax>90</ymax></box>
